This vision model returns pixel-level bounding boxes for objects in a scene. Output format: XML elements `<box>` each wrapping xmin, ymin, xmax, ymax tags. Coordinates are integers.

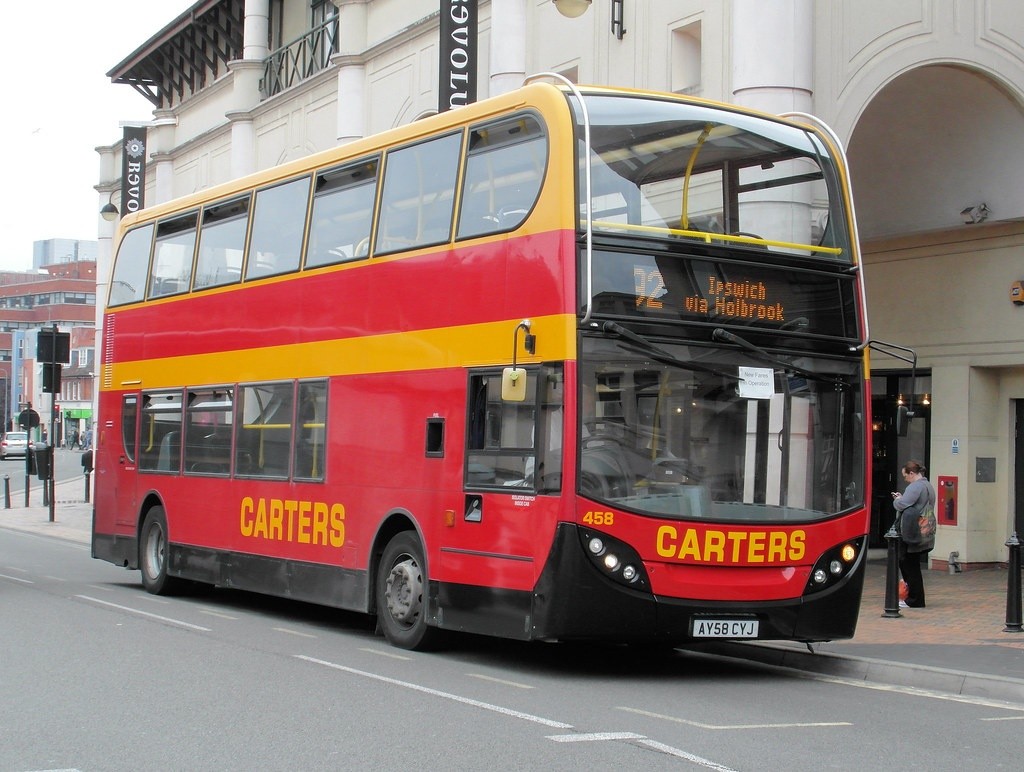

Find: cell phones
<box><xmin>891</xmin><ymin>492</ymin><xmax>898</xmax><ymax>497</ymax></box>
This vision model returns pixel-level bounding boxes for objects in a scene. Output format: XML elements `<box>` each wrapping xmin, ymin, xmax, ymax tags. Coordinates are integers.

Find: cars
<box><xmin>0</xmin><ymin>431</ymin><xmax>35</xmax><ymax>460</ymax></box>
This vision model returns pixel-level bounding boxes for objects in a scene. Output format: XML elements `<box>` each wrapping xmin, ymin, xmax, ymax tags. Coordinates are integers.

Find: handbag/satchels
<box><xmin>894</xmin><ymin>506</ymin><xmax>922</xmax><ymax>544</ymax></box>
<box><xmin>899</xmin><ymin>579</ymin><xmax>909</xmax><ymax>600</ymax></box>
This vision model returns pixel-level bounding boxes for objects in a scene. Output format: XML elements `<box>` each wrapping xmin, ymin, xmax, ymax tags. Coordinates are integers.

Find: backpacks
<box><xmin>918</xmin><ymin>503</ymin><xmax>937</xmax><ymax>537</ymax></box>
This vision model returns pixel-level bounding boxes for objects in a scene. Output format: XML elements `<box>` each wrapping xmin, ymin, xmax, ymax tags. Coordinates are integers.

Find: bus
<box><xmin>89</xmin><ymin>72</ymin><xmax>917</xmax><ymax>657</ymax></box>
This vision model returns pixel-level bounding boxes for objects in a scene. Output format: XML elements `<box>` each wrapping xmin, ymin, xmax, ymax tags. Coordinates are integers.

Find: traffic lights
<box><xmin>54</xmin><ymin>403</ymin><xmax>59</xmax><ymax>420</ymax></box>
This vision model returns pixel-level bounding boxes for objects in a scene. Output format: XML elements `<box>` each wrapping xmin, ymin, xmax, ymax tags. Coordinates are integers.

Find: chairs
<box><xmin>159</xmin><ymin>206</ymin><xmax>531</xmax><ymax>296</ymax></box>
<box><xmin>158</xmin><ymin>430</ymin><xmax>275</xmax><ymax>475</ymax></box>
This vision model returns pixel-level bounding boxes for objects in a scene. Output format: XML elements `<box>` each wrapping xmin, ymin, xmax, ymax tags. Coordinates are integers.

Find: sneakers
<box><xmin>899</xmin><ymin>601</ymin><xmax>909</xmax><ymax>608</ymax></box>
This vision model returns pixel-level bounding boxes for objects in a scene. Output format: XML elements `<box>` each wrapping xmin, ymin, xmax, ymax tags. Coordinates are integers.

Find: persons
<box><xmin>69</xmin><ymin>427</ymin><xmax>91</xmax><ymax>451</ymax></box>
<box><xmin>892</xmin><ymin>462</ymin><xmax>937</xmax><ymax>608</ymax></box>
<box><xmin>41</xmin><ymin>429</ymin><xmax>47</xmax><ymax>442</ymax></box>
